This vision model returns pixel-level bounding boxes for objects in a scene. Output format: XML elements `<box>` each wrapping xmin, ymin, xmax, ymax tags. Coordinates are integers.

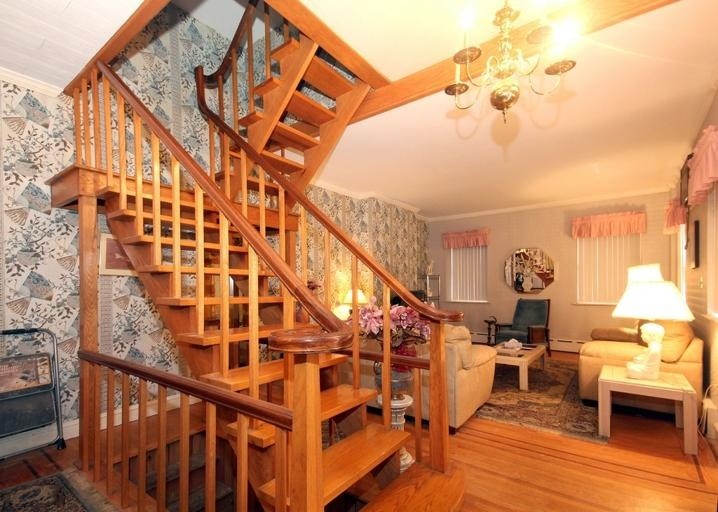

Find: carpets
<box><xmin>473</xmin><ymin>359</ymin><xmax>610</xmax><ymax>445</ymax></box>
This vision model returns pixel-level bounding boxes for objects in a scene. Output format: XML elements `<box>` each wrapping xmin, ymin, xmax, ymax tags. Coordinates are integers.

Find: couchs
<box><xmin>330</xmin><ymin>315</ymin><xmax>495</xmax><ymax>435</ymax></box>
<box><xmin>577</xmin><ymin>320</ymin><xmax>704</xmax><ymax>414</ymax></box>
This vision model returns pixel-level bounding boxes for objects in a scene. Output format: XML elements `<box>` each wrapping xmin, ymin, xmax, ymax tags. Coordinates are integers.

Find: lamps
<box><xmin>616</xmin><ymin>263</ymin><xmax>697</xmax><ymax>381</ymax></box>
<box><xmin>446</xmin><ymin>0</ymin><xmax>594</xmax><ymax>123</ymax></box>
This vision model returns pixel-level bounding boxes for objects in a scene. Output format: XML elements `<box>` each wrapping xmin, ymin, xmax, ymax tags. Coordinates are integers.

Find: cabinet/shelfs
<box><xmin>415</xmin><ymin>274</ymin><xmax>441</xmax><ymax>311</ymax></box>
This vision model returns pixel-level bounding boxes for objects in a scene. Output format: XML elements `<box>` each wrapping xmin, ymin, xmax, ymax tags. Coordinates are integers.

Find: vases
<box><xmin>373</xmin><ymin>341</ymin><xmax>413</xmax><ymax>400</ymax></box>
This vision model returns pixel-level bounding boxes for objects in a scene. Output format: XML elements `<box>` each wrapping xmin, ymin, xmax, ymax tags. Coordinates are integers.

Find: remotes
<box><xmin>522</xmin><ymin>343</ymin><xmax>538</xmax><ymax>348</ymax></box>
<box><xmin>521</xmin><ymin>347</ymin><xmax>532</xmax><ymax>350</ymax></box>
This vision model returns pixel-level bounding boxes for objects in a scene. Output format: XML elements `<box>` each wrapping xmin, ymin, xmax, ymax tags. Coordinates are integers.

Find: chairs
<box><xmin>492</xmin><ymin>297</ymin><xmax>549</xmax><ymax>365</ymax></box>
<box><xmin>0</xmin><ymin>328</ymin><xmax>66</xmax><ymax>461</ymax></box>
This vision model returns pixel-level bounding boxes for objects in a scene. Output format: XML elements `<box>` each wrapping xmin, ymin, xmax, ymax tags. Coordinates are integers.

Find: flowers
<box><xmin>346</xmin><ymin>294</ymin><xmax>432</xmax><ymax>347</ymax></box>
